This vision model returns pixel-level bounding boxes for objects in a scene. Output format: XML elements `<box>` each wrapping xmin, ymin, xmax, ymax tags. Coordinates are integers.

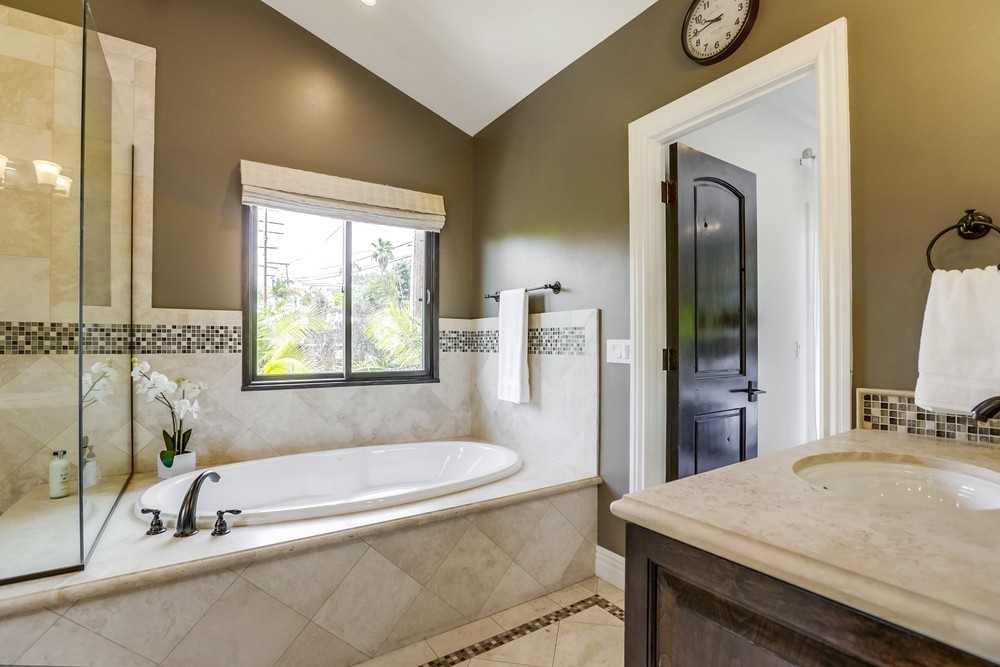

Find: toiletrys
<box><xmin>48</xmin><ymin>450</ymin><xmax>70</xmax><ymax>499</ymax></box>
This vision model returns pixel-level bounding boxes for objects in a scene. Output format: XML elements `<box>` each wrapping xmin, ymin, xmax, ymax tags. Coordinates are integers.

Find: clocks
<box><xmin>681</xmin><ymin>0</ymin><xmax>759</xmax><ymax>65</ymax></box>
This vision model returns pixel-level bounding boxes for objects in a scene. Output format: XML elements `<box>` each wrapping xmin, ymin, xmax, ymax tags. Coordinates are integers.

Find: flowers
<box><xmin>131</xmin><ymin>357</ymin><xmax>207</xmax><ymax>471</ymax></box>
<box><xmin>82</xmin><ymin>359</ymin><xmax>117</xmax><ymax>467</ymax></box>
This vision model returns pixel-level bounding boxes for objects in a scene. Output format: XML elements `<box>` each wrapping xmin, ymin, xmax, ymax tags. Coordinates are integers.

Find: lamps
<box><xmin>0</xmin><ymin>153</ymin><xmax>71</xmax><ymax>221</ymax></box>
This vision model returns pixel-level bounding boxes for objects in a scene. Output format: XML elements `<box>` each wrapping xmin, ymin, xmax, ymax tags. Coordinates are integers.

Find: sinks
<box><xmin>793</xmin><ymin>453</ymin><xmax>998</xmax><ymax>511</ymax></box>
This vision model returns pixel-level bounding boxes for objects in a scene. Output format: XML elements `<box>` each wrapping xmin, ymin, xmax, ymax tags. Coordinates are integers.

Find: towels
<box><xmin>496</xmin><ymin>287</ymin><xmax>531</xmax><ymax>404</ymax></box>
<box><xmin>913</xmin><ymin>264</ymin><xmax>999</xmax><ymax>415</ymax></box>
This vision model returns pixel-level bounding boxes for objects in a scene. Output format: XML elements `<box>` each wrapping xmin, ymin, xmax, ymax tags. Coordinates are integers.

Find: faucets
<box><xmin>970</xmin><ymin>396</ymin><xmax>1000</xmax><ymax>422</ymax></box>
<box><xmin>141</xmin><ymin>470</ymin><xmax>242</xmax><ymax>538</ymax></box>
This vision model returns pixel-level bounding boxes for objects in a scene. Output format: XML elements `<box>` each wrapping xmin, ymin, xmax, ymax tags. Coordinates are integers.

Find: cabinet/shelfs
<box><xmin>626</xmin><ymin>522</ymin><xmax>999</xmax><ymax>667</ymax></box>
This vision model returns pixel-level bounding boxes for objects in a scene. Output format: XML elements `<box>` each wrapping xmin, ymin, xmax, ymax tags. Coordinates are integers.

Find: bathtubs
<box><xmin>134</xmin><ymin>441</ymin><xmax>524</xmax><ymax>530</ymax></box>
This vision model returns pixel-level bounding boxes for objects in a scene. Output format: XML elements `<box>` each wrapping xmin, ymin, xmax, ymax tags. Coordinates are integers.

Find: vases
<box><xmin>83</xmin><ymin>457</ymin><xmax>102</xmax><ymax>489</ymax></box>
<box><xmin>156</xmin><ymin>450</ymin><xmax>196</xmax><ymax>480</ymax></box>
